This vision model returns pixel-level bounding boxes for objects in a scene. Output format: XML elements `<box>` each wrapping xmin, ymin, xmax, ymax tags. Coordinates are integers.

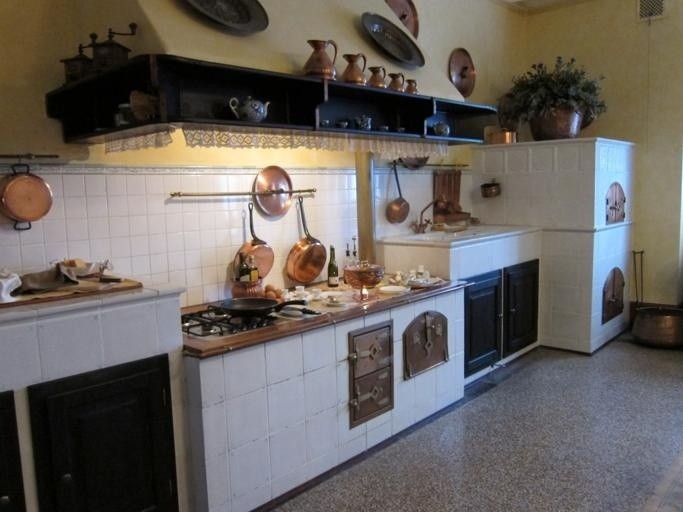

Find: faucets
<box><xmin>410</xmin><ymin>195</ymin><xmax>447</xmax><ymax>232</ymax></box>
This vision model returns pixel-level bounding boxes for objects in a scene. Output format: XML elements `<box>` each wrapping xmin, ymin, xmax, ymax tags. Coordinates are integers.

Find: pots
<box><xmin>233</xmin><ymin>203</ymin><xmax>274</xmax><ymax>280</ymax></box>
<box><xmin>286</xmin><ymin>196</ymin><xmax>327</xmax><ymax>283</ymax></box>
<box><xmin>633</xmin><ymin>306</ymin><xmax>683</xmax><ymax>348</ymax></box>
<box><xmin>220</xmin><ymin>297</ymin><xmax>306</xmax><ymax>316</ymax></box>
<box><xmin>387</xmin><ymin>160</ymin><xmax>410</xmax><ymax>224</ymax></box>
<box><xmin>0</xmin><ymin>164</ymin><xmax>53</xmax><ymax>231</ymax></box>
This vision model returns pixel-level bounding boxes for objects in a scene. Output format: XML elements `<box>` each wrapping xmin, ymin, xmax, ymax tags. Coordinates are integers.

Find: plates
<box><xmin>322</xmin><ymin>291</ymin><xmax>344</xmax><ymax>297</ymax></box>
<box><xmin>361</xmin><ymin>11</ymin><xmax>429</xmax><ymax>68</ymax></box>
<box><xmin>179</xmin><ymin>1</ymin><xmax>270</xmax><ymax>33</ymax></box>
<box><xmin>377</xmin><ymin>284</ymin><xmax>413</xmax><ymax>293</ymax></box>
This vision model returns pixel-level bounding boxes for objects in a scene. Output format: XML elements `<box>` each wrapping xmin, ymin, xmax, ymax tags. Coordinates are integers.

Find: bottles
<box><xmin>251</xmin><ymin>255</ymin><xmax>259</xmax><ymax>282</ymax></box>
<box><xmin>236</xmin><ymin>251</ymin><xmax>250</xmax><ymax>283</ymax></box>
<box><xmin>327</xmin><ymin>246</ymin><xmax>340</xmax><ymax>287</ymax></box>
<box><xmin>352</xmin><ymin>243</ymin><xmax>360</xmax><ymax>267</ymax></box>
<box><xmin>345</xmin><ymin>243</ymin><xmax>352</xmax><ymax>267</ymax></box>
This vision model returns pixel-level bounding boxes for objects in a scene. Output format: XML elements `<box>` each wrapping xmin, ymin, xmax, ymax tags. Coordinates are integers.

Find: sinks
<box><xmin>403</xmin><ymin>226</ymin><xmax>513</xmax><ymax>243</ymax></box>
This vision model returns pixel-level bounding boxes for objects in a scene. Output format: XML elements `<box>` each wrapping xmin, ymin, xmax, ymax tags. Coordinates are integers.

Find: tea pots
<box><xmin>228</xmin><ymin>96</ymin><xmax>271</xmax><ymax>122</ymax></box>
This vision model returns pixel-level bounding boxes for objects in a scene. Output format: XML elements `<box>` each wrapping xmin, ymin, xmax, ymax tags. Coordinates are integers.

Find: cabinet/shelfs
<box><xmin>23</xmin><ymin>351</ymin><xmax>180</xmax><ymax>507</ymax></box>
<box><xmin>504</xmin><ymin>258</ymin><xmax>545</xmax><ymax>356</ymax></box>
<box><xmin>472</xmin><ymin>137</ymin><xmax>635</xmax><ymax>356</ymax></box>
<box><xmin>45</xmin><ymin>53</ymin><xmax>496</xmax><ymax>147</ymax></box>
<box><xmin>453</xmin><ymin>268</ymin><xmax>504</xmax><ymax>377</ymax></box>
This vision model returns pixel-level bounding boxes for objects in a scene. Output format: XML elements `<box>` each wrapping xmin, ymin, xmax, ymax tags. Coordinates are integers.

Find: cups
<box><xmin>312</xmin><ymin>289</ymin><xmax>322</xmax><ymax>298</ymax></box>
<box><xmin>376</xmin><ymin>124</ymin><xmax>387</xmax><ymax>131</ymax></box>
<box><xmin>321</xmin><ymin>119</ymin><xmax>332</xmax><ymax>127</ymax></box>
<box><xmin>395</xmin><ymin>127</ymin><xmax>406</xmax><ymax>133</ymax></box>
<box><xmin>335</xmin><ymin>121</ymin><xmax>347</xmax><ymax>128</ymax></box>
<box><xmin>296</xmin><ymin>286</ymin><xmax>304</xmax><ymax>296</ymax></box>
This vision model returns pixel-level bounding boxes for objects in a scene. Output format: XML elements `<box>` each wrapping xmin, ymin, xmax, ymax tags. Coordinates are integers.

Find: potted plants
<box><xmin>496</xmin><ymin>58</ymin><xmax>611</xmax><ymax>142</ymax></box>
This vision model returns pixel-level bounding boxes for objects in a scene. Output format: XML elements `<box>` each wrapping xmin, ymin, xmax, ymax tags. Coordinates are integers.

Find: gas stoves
<box><xmin>181</xmin><ymin>307</ymin><xmax>276</xmax><ymax>338</ymax></box>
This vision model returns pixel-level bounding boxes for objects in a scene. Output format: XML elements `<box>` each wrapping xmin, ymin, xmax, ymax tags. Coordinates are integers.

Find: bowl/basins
<box><xmin>343</xmin><ymin>264</ymin><xmax>384</xmax><ymax>288</ymax></box>
<box><xmin>480</xmin><ymin>183</ymin><xmax>500</xmax><ymax>199</ymax></box>
